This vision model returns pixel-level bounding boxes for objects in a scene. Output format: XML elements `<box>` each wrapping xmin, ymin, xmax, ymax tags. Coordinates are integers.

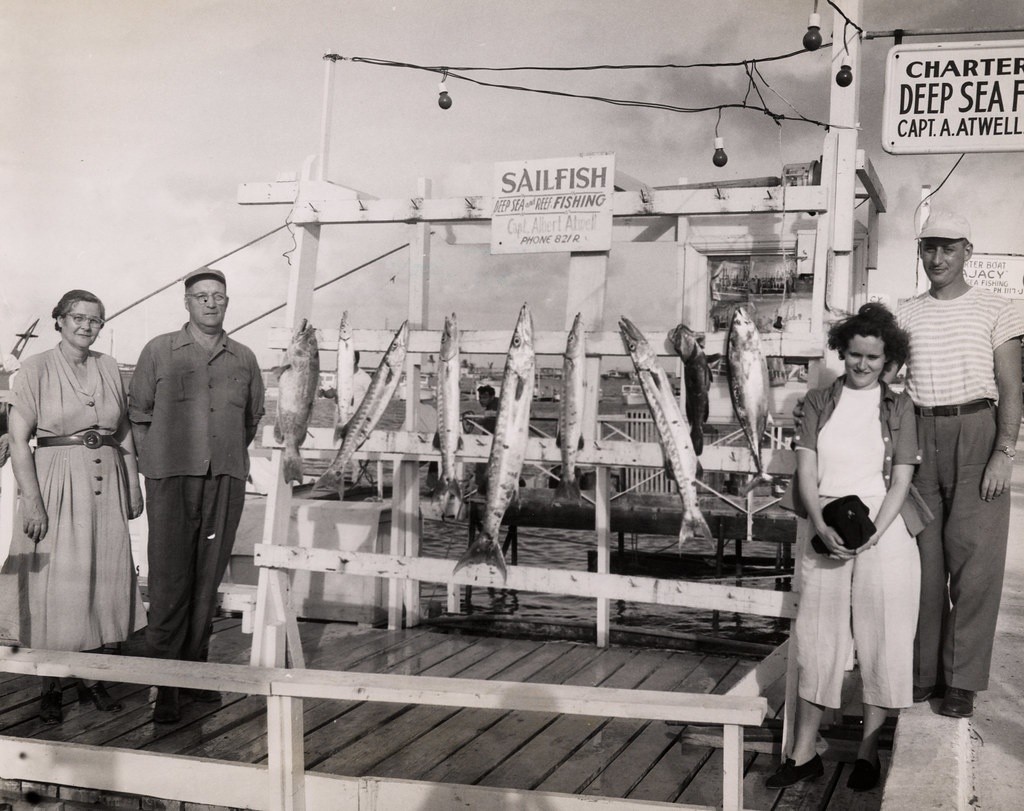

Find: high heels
<box><xmin>75</xmin><ymin>678</ymin><xmax>123</xmax><ymax>713</ymax></box>
<box><xmin>38</xmin><ymin>682</ymin><xmax>64</xmax><ymax>724</ymax></box>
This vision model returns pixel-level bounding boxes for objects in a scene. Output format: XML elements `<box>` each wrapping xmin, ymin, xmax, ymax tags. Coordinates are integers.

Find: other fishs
<box><xmin>272</xmin><ymin>301</ymin><xmax>770</xmax><ymax>584</ymax></box>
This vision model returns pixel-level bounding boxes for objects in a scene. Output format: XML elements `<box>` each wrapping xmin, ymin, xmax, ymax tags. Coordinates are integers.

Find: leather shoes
<box><xmin>939</xmin><ymin>686</ymin><xmax>975</xmax><ymax>717</ymax></box>
<box><xmin>184</xmin><ymin>688</ymin><xmax>223</xmax><ymax>703</ymax></box>
<box><xmin>912</xmin><ymin>685</ymin><xmax>936</xmax><ymax>702</ymax></box>
<box><xmin>153</xmin><ymin>685</ymin><xmax>182</xmax><ymax>724</ymax></box>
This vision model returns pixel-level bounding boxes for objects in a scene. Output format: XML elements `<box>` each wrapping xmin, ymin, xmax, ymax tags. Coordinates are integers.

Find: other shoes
<box><xmin>765</xmin><ymin>752</ymin><xmax>824</xmax><ymax>789</ymax></box>
<box><xmin>847</xmin><ymin>758</ymin><xmax>882</xmax><ymax>788</ymax></box>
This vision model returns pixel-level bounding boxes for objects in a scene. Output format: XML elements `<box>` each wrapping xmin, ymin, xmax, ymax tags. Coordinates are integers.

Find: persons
<box><xmin>127</xmin><ymin>268</ymin><xmax>266</xmax><ymax>725</ymax></box>
<box><xmin>475</xmin><ymin>386</ymin><xmax>526</xmax><ymax>492</ymax></box>
<box><xmin>0</xmin><ymin>290</ymin><xmax>149</xmax><ymax>725</ymax></box>
<box><xmin>882</xmin><ymin>212</ymin><xmax>1024</xmax><ymax>718</ymax></box>
<box><xmin>318</xmin><ymin>350</ymin><xmax>372</xmax><ymax>424</ymax></box>
<box><xmin>763</xmin><ymin>302</ymin><xmax>935</xmax><ymax>793</ymax></box>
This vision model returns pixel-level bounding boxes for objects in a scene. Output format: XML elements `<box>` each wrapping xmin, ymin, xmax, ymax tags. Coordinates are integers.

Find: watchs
<box><xmin>994</xmin><ymin>445</ymin><xmax>1016</xmax><ymax>457</ymax></box>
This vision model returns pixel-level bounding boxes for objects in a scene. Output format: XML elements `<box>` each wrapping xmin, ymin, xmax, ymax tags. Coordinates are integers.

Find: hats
<box><xmin>914</xmin><ymin>208</ymin><xmax>971</xmax><ymax>244</ymax></box>
<box><xmin>182</xmin><ymin>267</ymin><xmax>226</xmax><ymax>285</ymax></box>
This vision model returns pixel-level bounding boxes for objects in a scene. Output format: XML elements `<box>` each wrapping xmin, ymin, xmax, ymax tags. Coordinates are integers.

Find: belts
<box><xmin>914</xmin><ymin>399</ymin><xmax>994</xmax><ymax>417</ymax></box>
<box><xmin>37</xmin><ymin>430</ymin><xmax>122</xmax><ymax>447</ymax></box>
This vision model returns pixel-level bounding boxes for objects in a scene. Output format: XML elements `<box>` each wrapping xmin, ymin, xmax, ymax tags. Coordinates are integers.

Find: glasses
<box><xmin>183</xmin><ymin>292</ymin><xmax>227</xmax><ymax>306</ymax></box>
<box><xmin>64</xmin><ymin>312</ymin><xmax>105</xmax><ymax>328</ymax></box>
